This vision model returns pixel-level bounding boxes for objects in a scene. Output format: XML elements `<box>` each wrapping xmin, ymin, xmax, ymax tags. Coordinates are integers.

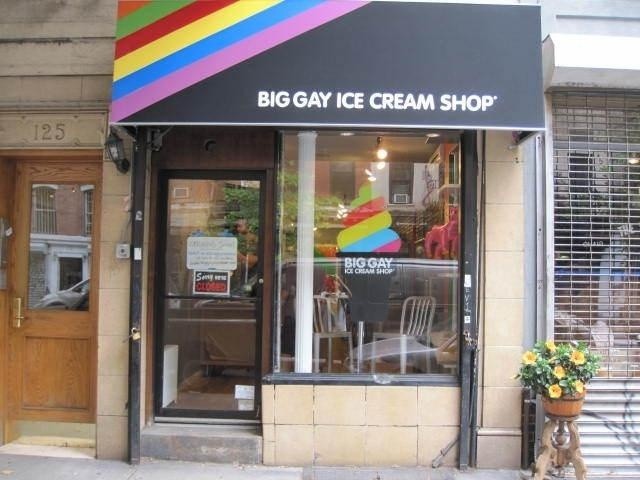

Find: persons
<box><xmin>422</xmin><ymin>165</ymin><xmax>433</xmax><ymax>205</ymax></box>
<box><xmin>237</xmin><ymin>218</ymin><xmax>259</xmax><ymax>285</ymax></box>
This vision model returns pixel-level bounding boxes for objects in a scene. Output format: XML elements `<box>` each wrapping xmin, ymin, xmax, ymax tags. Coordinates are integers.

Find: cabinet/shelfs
<box><xmin>530</xmin><ymin>412</ymin><xmax>588</xmax><ymax>480</ymax></box>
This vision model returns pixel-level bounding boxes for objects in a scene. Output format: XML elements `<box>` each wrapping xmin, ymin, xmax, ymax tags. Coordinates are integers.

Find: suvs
<box><xmin>32</xmin><ymin>270</ymin><xmax>183</xmax><ymax>309</ymax></box>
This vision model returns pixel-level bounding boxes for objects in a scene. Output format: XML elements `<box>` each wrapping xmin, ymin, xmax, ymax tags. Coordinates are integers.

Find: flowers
<box><xmin>513</xmin><ymin>339</ymin><xmax>604</xmax><ymax>398</ymax></box>
<box><xmin>325</xmin><ymin>272</ymin><xmax>353</xmax><ymax>297</ymax></box>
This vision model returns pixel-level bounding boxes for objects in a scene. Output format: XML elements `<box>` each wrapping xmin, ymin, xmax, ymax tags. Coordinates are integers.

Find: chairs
<box><xmin>369</xmin><ymin>295</ymin><xmax>437</xmax><ymax>374</ymax></box>
<box><xmin>200</xmin><ymin>302</ymin><xmax>260</xmax><ymax>376</ymax></box>
<box><xmin>313</xmin><ymin>295</ymin><xmax>355</xmax><ymax>373</ymax></box>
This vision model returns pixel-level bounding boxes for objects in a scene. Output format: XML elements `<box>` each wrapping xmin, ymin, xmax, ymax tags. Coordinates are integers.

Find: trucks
<box><xmin>178</xmin><ymin>254</ymin><xmax>460</xmax><ymax>389</ymax></box>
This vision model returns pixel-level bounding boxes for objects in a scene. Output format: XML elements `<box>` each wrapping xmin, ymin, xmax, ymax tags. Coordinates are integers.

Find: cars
<box><xmin>168</xmin><ymin>292</ymin><xmax>181</xmax><ymax>310</ymax></box>
<box><xmin>71</xmin><ymin>292</ymin><xmax>90</xmax><ymax>311</ymax></box>
<box><xmin>344</xmin><ymin>308</ymin><xmax>617</xmax><ymax>378</ymax></box>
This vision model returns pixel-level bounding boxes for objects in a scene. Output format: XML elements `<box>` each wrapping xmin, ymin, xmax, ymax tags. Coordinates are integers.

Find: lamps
<box><xmin>104</xmin><ymin>127</ymin><xmax>130</xmax><ymax>174</ymax></box>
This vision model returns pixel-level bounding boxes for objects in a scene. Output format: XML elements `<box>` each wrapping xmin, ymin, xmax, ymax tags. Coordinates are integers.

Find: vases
<box><xmin>541</xmin><ymin>388</ymin><xmax>586</xmax><ymax>416</ymax></box>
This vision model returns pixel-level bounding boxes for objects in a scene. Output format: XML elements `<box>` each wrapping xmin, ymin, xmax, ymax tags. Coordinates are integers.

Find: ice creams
<box><xmin>336</xmin><ymin>177</ymin><xmax>401</xmax><ymax>325</ymax></box>
